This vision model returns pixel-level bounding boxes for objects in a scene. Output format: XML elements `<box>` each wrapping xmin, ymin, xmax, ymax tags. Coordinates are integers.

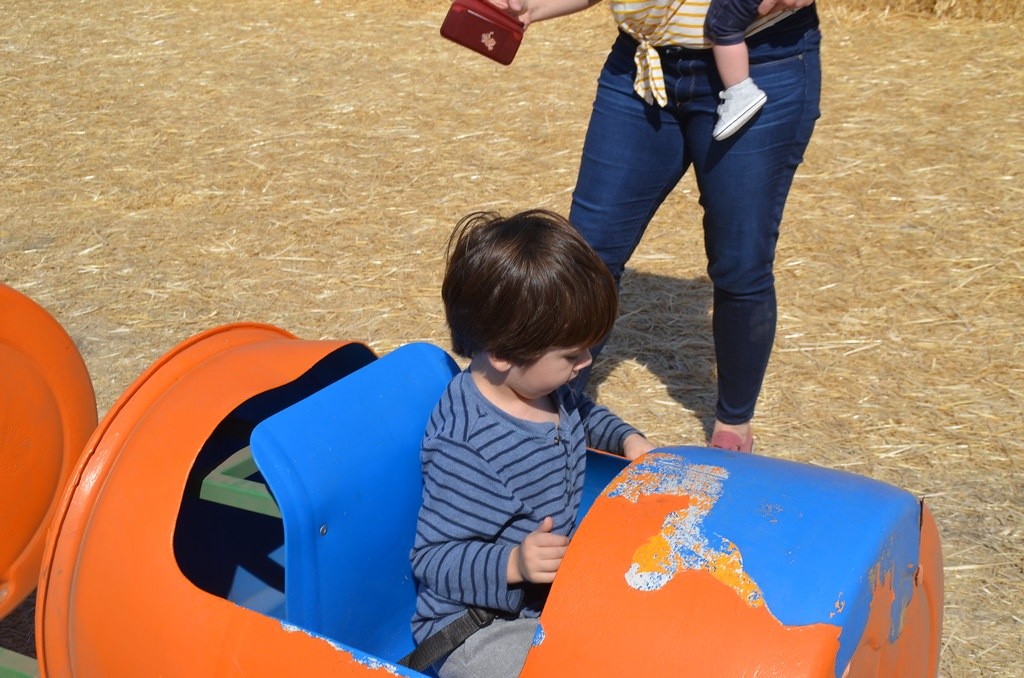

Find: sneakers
<box><xmin>714</xmin><ymin>78</ymin><xmax>768</xmax><ymax>141</ymax></box>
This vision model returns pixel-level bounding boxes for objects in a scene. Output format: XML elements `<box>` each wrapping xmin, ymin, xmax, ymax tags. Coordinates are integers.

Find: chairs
<box><xmin>249</xmin><ymin>341</ymin><xmax>441</xmax><ymax>678</ymax></box>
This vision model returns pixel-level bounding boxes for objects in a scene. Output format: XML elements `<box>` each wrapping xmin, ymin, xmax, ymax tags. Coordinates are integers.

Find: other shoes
<box><xmin>709</xmin><ymin>430</ymin><xmax>754</xmax><ymax>454</ymax></box>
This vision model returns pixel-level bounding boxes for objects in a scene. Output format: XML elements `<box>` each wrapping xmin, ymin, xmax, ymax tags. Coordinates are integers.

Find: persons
<box><xmin>705</xmin><ymin>0</ymin><xmax>767</xmax><ymax>140</ymax></box>
<box><xmin>409</xmin><ymin>209</ymin><xmax>658</xmax><ymax>678</ymax></box>
<box><xmin>492</xmin><ymin>0</ymin><xmax>821</xmax><ymax>454</ymax></box>
